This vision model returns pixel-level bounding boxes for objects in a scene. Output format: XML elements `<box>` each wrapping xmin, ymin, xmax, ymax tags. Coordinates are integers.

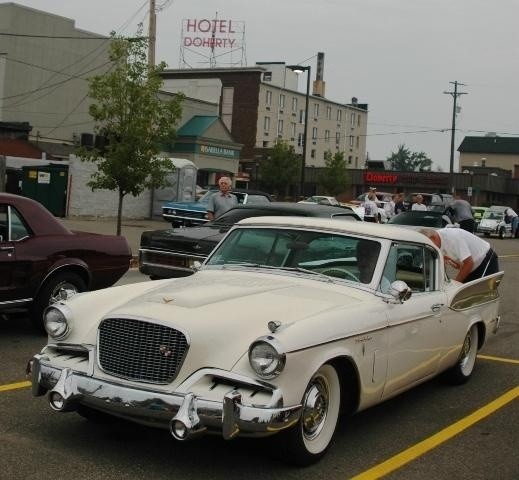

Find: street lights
<box><xmin>286</xmin><ymin>65</ymin><xmax>311</xmax><ymax>196</ymax></box>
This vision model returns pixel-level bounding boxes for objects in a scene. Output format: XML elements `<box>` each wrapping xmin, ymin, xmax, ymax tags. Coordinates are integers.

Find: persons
<box><xmin>505</xmin><ymin>208</ymin><xmax>519</xmax><ymax>238</ymax></box>
<box><xmin>416</xmin><ymin>227</ymin><xmax>500</xmax><ymax>283</ymax></box>
<box><xmin>207</xmin><ymin>176</ymin><xmax>237</xmax><ymax>221</ymax></box>
<box><xmin>363</xmin><ymin>186</ymin><xmax>443</xmax><ymax>223</ymax></box>
<box><xmin>344</xmin><ymin>237</ymin><xmax>391</xmax><ymax>296</ymax></box>
<box><xmin>449</xmin><ymin>192</ymin><xmax>475</xmax><ymax>233</ymax></box>
